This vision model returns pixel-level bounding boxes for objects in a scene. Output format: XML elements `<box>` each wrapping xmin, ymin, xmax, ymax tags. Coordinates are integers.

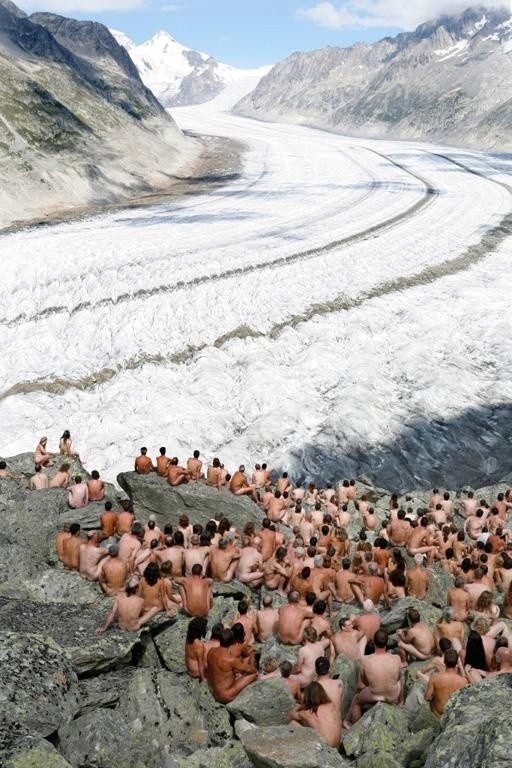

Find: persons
<box><xmin>49</xmin><ymin>463</ymin><xmax>71</xmax><ymax>487</ymax></box>
<box><xmin>55</xmin><ymin>447</ymin><xmax>511</xmax><ymax>749</ymax></box>
<box><xmin>1</xmin><ymin>461</ymin><xmax>25</xmax><ymax>480</ymax></box>
<box><xmin>32</xmin><ymin>436</ymin><xmax>56</xmax><ymax>467</ymax></box>
<box><xmin>58</xmin><ymin>430</ymin><xmax>88</xmax><ymax>465</ymax></box>
<box><xmin>30</xmin><ymin>464</ymin><xmax>49</xmax><ymax>490</ymax></box>
